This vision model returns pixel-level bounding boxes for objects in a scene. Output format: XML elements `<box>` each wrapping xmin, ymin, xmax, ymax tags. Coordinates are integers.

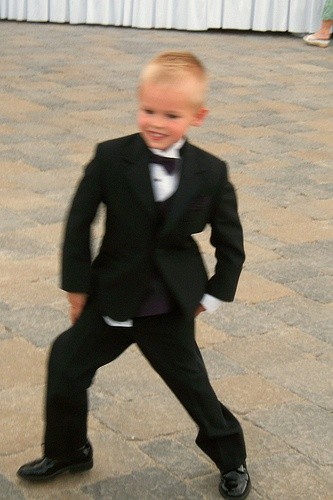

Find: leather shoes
<box><xmin>217</xmin><ymin>463</ymin><xmax>252</xmax><ymax>500</ymax></box>
<box><xmin>16</xmin><ymin>442</ymin><xmax>94</xmax><ymax>482</ymax></box>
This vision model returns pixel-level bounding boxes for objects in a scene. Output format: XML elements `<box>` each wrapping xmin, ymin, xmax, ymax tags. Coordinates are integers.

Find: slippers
<box><xmin>303</xmin><ymin>34</ymin><xmax>331</xmax><ymax>48</ymax></box>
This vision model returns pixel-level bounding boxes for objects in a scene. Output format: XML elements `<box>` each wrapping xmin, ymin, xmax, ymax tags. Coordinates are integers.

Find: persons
<box><xmin>303</xmin><ymin>0</ymin><xmax>333</xmax><ymax>48</ymax></box>
<box><xmin>16</xmin><ymin>50</ymin><xmax>252</xmax><ymax>500</ymax></box>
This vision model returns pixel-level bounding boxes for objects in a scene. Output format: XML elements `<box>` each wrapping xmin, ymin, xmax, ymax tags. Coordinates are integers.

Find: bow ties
<box><xmin>135</xmin><ymin>140</ymin><xmax>180</xmax><ymax>171</ymax></box>
